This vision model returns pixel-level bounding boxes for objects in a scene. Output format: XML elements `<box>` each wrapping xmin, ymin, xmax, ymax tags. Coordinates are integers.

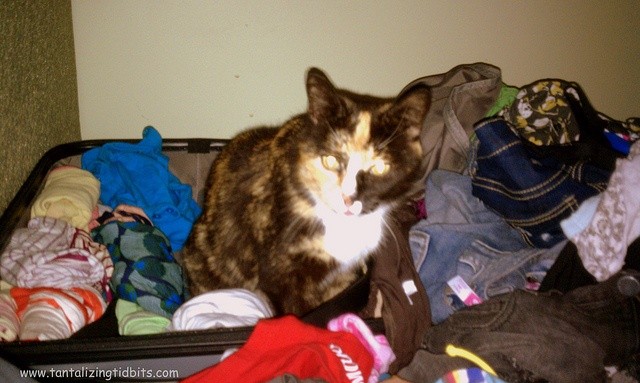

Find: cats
<box><xmin>180</xmin><ymin>65</ymin><xmax>433</xmax><ymax>315</ymax></box>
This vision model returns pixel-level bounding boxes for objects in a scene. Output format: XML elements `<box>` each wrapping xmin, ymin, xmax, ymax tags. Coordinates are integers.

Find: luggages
<box><xmin>0</xmin><ymin>138</ymin><xmax>384</xmax><ymax>383</ymax></box>
